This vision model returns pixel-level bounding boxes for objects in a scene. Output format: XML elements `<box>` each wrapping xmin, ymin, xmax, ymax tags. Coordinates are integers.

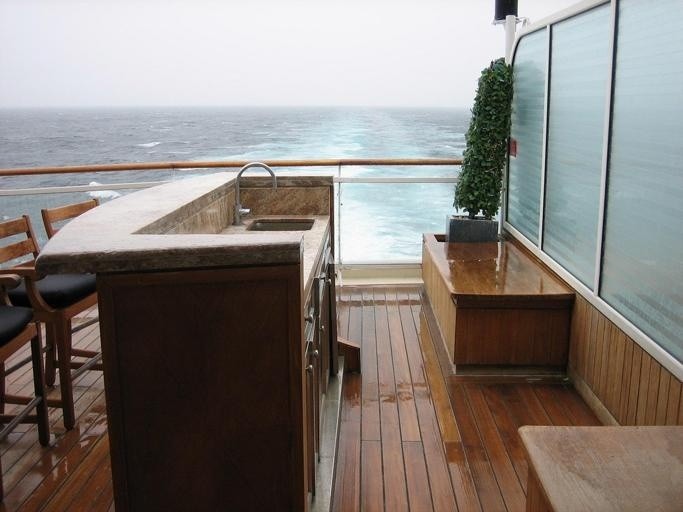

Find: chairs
<box><xmin>1</xmin><ymin>196</ymin><xmax>100</xmax><ymax>447</ymax></box>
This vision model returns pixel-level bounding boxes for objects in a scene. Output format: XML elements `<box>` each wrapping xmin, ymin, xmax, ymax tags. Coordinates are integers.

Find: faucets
<box><xmin>233</xmin><ymin>162</ymin><xmax>277</xmax><ymax>226</ymax></box>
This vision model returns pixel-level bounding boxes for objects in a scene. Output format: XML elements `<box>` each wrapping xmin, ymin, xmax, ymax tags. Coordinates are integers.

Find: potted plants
<box><xmin>446</xmin><ymin>58</ymin><xmax>513</xmax><ymax>241</ymax></box>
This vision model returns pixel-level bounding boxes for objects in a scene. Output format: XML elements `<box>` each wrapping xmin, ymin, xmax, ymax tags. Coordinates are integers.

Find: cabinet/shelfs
<box><xmin>302</xmin><ymin>226</ymin><xmax>337</xmax><ymax>506</ymax></box>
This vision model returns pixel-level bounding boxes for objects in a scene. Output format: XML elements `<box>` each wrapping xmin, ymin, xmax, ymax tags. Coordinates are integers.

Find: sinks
<box><xmin>246</xmin><ymin>219</ymin><xmax>315</xmax><ymax>229</ymax></box>
